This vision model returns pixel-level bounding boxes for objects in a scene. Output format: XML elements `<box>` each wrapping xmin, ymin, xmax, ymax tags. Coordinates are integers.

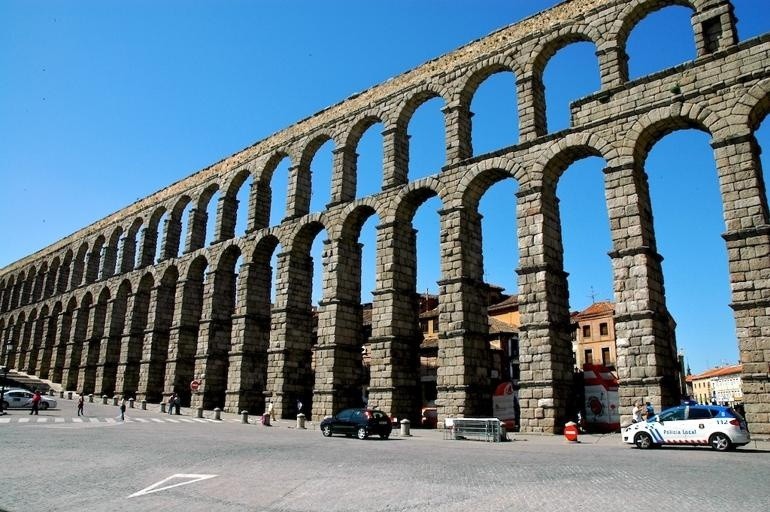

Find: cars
<box><xmin>2</xmin><ymin>390</ymin><xmax>58</xmax><ymax>411</ymax></box>
<box><xmin>620</xmin><ymin>399</ymin><xmax>751</xmax><ymax>452</ymax></box>
<box><xmin>318</xmin><ymin>408</ymin><xmax>393</xmax><ymax>440</ymax></box>
<box><xmin>0</xmin><ymin>386</ymin><xmax>26</xmax><ymax>398</ymax></box>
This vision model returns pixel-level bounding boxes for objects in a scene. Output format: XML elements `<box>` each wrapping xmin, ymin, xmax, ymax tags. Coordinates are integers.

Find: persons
<box><xmin>167</xmin><ymin>393</ymin><xmax>174</xmax><ymax>415</ymax></box>
<box><xmin>269</xmin><ymin>401</ymin><xmax>276</xmax><ymax>421</ymax></box>
<box><xmin>29</xmin><ymin>390</ymin><xmax>41</xmax><ymax>415</ymax></box>
<box><xmin>174</xmin><ymin>393</ymin><xmax>180</xmax><ymax>415</ymax></box>
<box><xmin>632</xmin><ymin>400</ymin><xmax>644</xmax><ymax>422</ymax></box>
<box><xmin>646</xmin><ymin>402</ymin><xmax>653</xmax><ymax>419</ymax></box>
<box><xmin>120</xmin><ymin>399</ymin><xmax>126</xmax><ymax>420</ymax></box>
<box><xmin>296</xmin><ymin>399</ymin><xmax>303</xmax><ymax>413</ymax></box>
<box><xmin>77</xmin><ymin>393</ymin><xmax>84</xmax><ymax>416</ymax></box>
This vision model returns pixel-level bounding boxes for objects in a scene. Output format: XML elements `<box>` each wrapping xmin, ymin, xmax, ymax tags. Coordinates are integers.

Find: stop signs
<box><xmin>190</xmin><ymin>380</ymin><xmax>199</xmax><ymax>390</ymax></box>
<box><xmin>564</xmin><ymin>426</ymin><xmax>578</xmax><ymax>441</ymax></box>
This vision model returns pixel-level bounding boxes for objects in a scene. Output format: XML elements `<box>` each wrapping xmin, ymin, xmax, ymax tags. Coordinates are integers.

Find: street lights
<box><xmin>0</xmin><ymin>339</ymin><xmax>14</xmax><ymax>416</ymax></box>
